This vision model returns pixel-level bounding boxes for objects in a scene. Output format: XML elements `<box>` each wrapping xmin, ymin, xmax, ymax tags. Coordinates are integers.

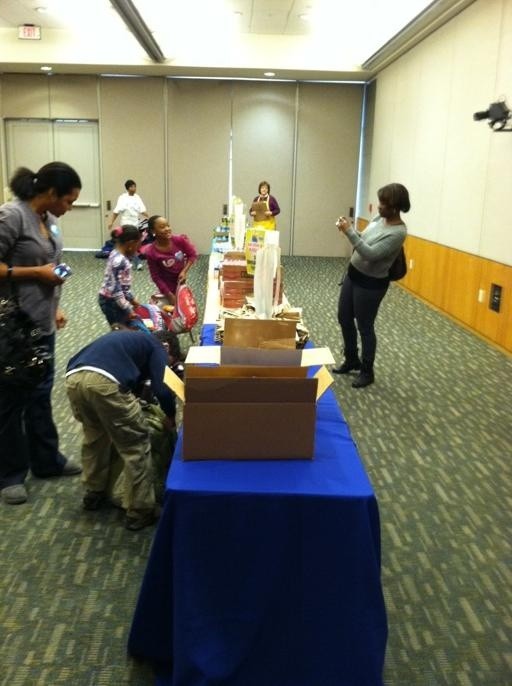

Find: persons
<box><xmin>112</xmin><ymin>321</ymin><xmax>184</xmax><ymax>408</ymax></box>
<box><xmin>249</xmin><ymin>182</ymin><xmax>280</xmax><ymax>231</ymax></box>
<box><xmin>0</xmin><ymin>161</ymin><xmax>82</xmax><ymax>504</ymax></box>
<box><xmin>107</xmin><ymin>180</ymin><xmax>152</xmax><ymax>271</ymax></box>
<box><xmin>63</xmin><ymin>329</ymin><xmax>180</xmax><ymax>531</ymax></box>
<box><xmin>332</xmin><ymin>183</ymin><xmax>410</xmax><ymax>389</ymax></box>
<box><xmin>96</xmin><ymin>224</ymin><xmax>142</xmax><ymax>329</ymax></box>
<box><xmin>143</xmin><ymin>215</ymin><xmax>197</xmax><ymax>306</ymax></box>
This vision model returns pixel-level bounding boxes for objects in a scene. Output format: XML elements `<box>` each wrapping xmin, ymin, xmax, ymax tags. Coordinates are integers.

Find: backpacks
<box><xmin>128</xmin><ymin>303</ymin><xmax>174</xmax><ymax>347</ymax></box>
<box><xmin>152</xmin><ymin>281</ymin><xmax>199</xmax><ymax>337</ymax></box>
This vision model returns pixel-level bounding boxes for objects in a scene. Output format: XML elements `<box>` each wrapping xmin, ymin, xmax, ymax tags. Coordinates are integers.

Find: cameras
<box><xmin>335</xmin><ymin>216</ymin><xmax>347</xmax><ymax>228</ymax></box>
<box><xmin>53</xmin><ymin>262</ymin><xmax>73</xmax><ymax>278</ymax></box>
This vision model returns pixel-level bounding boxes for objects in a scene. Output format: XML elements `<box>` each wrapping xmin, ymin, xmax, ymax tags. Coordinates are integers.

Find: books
<box><xmin>251</xmin><ymin>202</ymin><xmax>270</xmax><ymax>223</ymax></box>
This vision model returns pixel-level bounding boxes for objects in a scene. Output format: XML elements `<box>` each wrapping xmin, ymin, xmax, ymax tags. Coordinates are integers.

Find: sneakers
<box><xmin>121</xmin><ymin>503</ymin><xmax>162</xmax><ymax>531</ymax></box>
<box><xmin>82</xmin><ymin>490</ymin><xmax>123</xmax><ymax>513</ymax></box>
<box><xmin>0</xmin><ymin>482</ymin><xmax>30</xmax><ymax>507</ymax></box>
<box><xmin>32</xmin><ymin>457</ymin><xmax>83</xmax><ymax>480</ymax></box>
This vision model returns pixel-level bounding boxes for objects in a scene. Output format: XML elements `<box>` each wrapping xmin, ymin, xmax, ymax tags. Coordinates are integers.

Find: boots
<box><xmin>351</xmin><ymin>359</ymin><xmax>375</xmax><ymax>388</ymax></box>
<box><xmin>332</xmin><ymin>347</ymin><xmax>362</xmax><ymax>375</ymax></box>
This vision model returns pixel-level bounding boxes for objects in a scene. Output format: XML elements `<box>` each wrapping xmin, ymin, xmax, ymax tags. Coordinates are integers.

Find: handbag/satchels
<box><xmin>378</xmin><ymin>235</ymin><xmax>407</xmax><ymax>284</ymax></box>
<box><xmin>1</xmin><ymin>265</ymin><xmax>53</xmax><ymax>377</ymax></box>
